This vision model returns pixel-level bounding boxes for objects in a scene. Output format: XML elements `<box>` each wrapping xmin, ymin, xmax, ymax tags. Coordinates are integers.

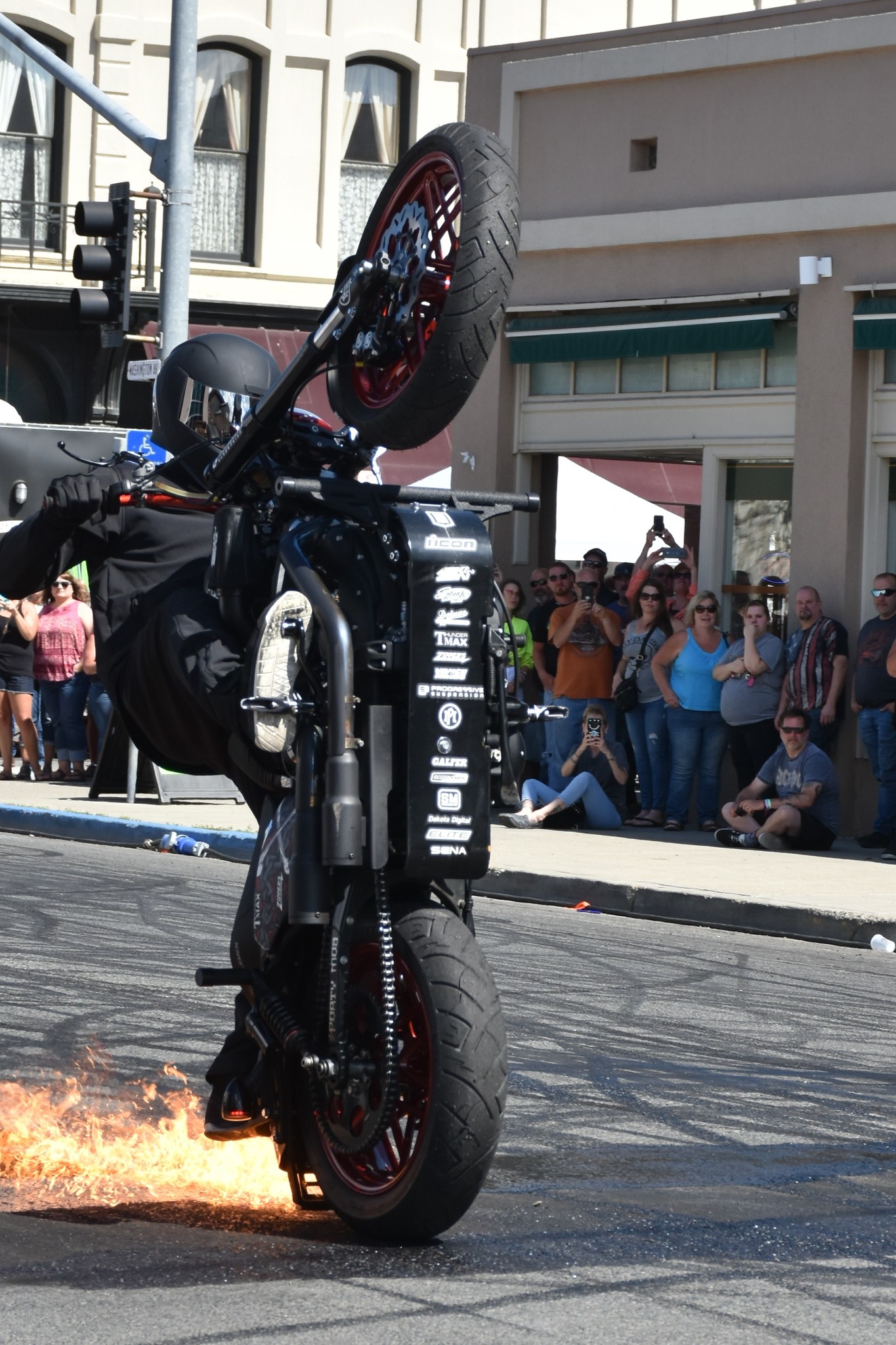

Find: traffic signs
<box><xmin>127</xmin><ymin>359</ymin><xmax>161</xmax><ymax>381</ymax></box>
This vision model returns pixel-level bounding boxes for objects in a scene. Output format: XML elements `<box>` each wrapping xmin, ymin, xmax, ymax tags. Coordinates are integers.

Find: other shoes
<box><xmin>663</xmin><ymin>820</ymin><xmax>683</xmax><ymax>832</ymax></box>
<box><xmin>880</xmin><ymin>846</ymin><xmax>896</xmax><ymax>860</ymax></box>
<box><xmin>699</xmin><ymin>821</ymin><xmax>719</xmax><ymax>831</ymax></box>
<box><xmin>498</xmin><ymin>813</ymin><xmax>543</xmax><ymax>829</ymax></box>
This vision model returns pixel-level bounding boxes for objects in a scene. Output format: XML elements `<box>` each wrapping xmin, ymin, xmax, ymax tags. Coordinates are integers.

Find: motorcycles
<box><xmin>46</xmin><ymin>124</ymin><xmax>543</xmax><ymax>1247</ymax></box>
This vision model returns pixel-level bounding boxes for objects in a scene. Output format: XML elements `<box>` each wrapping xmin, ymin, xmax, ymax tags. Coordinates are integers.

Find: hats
<box><xmin>584</xmin><ymin>548</ymin><xmax>607</xmax><ymax>562</ymax></box>
<box><xmin>614</xmin><ymin>562</ymin><xmax>635</xmax><ymax>576</ymax></box>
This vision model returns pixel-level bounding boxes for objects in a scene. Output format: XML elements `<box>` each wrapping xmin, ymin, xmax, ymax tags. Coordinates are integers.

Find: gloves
<box><xmin>46</xmin><ymin>474</ymin><xmax>110</xmax><ymax>526</ymax></box>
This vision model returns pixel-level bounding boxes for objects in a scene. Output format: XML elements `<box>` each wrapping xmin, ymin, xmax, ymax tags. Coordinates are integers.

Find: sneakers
<box><xmin>244</xmin><ymin>589</ymin><xmax>317</xmax><ymax>753</ymax></box>
<box><xmin>758</xmin><ymin>832</ymin><xmax>786</xmax><ymax>852</ymax></box>
<box><xmin>715</xmin><ymin>828</ymin><xmax>750</xmax><ymax>848</ymax></box>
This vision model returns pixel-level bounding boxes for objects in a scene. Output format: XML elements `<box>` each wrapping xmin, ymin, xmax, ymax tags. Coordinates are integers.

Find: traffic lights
<box><xmin>68</xmin><ymin>180</ymin><xmax>136</xmax><ymax>348</ymax></box>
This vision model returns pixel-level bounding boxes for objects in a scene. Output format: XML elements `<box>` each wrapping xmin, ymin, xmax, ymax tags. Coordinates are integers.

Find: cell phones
<box><xmin>588</xmin><ymin>718</ymin><xmax>599</xmax><ymax>741</ymax></box>
<box><xmin>582</xmin><ymin>585</ymin><xmax>594</xmax><ymax>605</ymax></box>
<box><xmin>662</xmin><ymin>547</ymin><xmax>687</xmax><ymax>559</ymax></box>
<box><xmin>653</xmin><ymin>516</ymin><xmax>664</xmax><ymax>536</ymax></box>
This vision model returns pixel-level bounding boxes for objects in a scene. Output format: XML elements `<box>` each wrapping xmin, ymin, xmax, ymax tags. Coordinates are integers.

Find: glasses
<box><xmin>52</xmin><ymin>581</ymin><xmax>72</xmax><ymax>588</ymax></box>
<box><xmin>871</xmin><ymin>588</ymin><xmax>896</xmax><ymax>597</ymax></box>
<box><xmin>694</xmin><ymin>604</ymin><xmax>717</xmax><ymax>613</ymax></box>
<box><xmin>639</xmin><ymin>592</ymin><xmax>661</xmax><ymax>602</ymax></box>
<box><xmin>583</xmin><ymin>560</ymin><xmax>603</xmax><ymax>569</ymax></box>
<box><xmin>673</xmin><ymin>571</ymin><xmax>691</xmax><ymax>579</ymax></box>
<box><xmin>549</xmin><ymin>572</ymin><xmax>569</xmax><ymax>582</ymax></box>
<box><xmin>781</xmin><ymin>726</ymin><xmax>805</xmax><ymax>734</ymax></box>
<box><xmin>655</xmin><ymin>571</ymin><xmax>674</xmax><ymax>580</ymax></box>
<box><xmin>574</xmin><ymin>581</ymin><xmax>599</xmax><ymax>589</ymax></box>
<box><xmin>529</xmin><ymin>578</ymin><xmax>547</xmax><ymax>587</ymax></box>
<box><xmin>503</xmin><ymin>590</ymin><xmax>522</xmax><ymax>598</ymax></box>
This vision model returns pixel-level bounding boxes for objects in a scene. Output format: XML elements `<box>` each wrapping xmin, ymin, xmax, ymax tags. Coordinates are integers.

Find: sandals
<box><xmin>0</xmin><ymin>763</ymin><xmax>97</xmax><ymax>782</ymax></box>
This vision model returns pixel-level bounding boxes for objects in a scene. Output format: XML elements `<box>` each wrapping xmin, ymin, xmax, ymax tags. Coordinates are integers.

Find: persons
<box><xmin>0</xmin><ymin>333</ymin><xmax>379</xmax><ymax>778</ymax></box>
<box><xmin>773</xmin><ymin>586</ymin><xmax>848</xmax><ymax>756</ymax></box>
<box><xmin>712</xmin><ymin>598</ymin><xmax>786</xmax><ymax>826</ymax></box>
<box><xmin>850</xmin><ymin>573</ymin><xmax>896</xmax><ymax>860</ymax></box>
<box><xmin>651</xmin><ymin>591</ymin><xmax>734</xmax><ymax>833</ymax></box>
<box><xmin>490</xmin><ymin>524</ymin><xmax>698</xmax><ymax>828</ymax></box>
<box><xmin>0</xmin><ymin>571</ymin><xmax>113</xmax><ymax>782</ymax></box>
<box><xmin>713</xmin><ymin>707</ymin><xmax>839</xmax><ymax>853</ymax></box>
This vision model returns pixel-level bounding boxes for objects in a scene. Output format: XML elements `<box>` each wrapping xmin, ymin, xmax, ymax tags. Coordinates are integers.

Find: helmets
<box><xmin>148</xmin><ymin>330</ymin><xmax>282</xmax><ymax>465</ymax></box>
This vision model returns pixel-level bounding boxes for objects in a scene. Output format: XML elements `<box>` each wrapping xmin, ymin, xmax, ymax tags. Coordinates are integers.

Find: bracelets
<box><xmin>574</xmin><ymin>753</ymin><xmax>581</xmax><ymax>758</ymax></box>
<box><xmin>763</xmin><ymin>798</ymin><xmax>771</xmax><ymax>809</ymax></box>
<box><xmin>569</xmin><ymin>756</ymin><xmax>578</xmax><ymax>764</ymax></box>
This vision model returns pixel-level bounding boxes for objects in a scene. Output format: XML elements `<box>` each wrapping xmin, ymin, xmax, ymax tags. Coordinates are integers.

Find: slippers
<box><xmin>624</xmin><ymin>816</ymin><xmax>662</xmax><ymax>828</ymax></box>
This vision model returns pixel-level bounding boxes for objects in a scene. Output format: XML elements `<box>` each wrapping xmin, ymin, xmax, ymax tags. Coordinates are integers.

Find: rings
<box><xmin>650</xmin><ymin>530</ymin><xmax>651</xmax><ymax>531</ymax></box>
<box><xmin>672</xmin><ymin>703</ymin><xmax>675</xmax><ymax>706</ymax></box>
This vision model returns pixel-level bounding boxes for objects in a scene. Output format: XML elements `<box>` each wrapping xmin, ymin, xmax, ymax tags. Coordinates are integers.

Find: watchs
<box><xmin>607</xmin><ymin>754</ymin><xmax>616</xmax><ymax>760</ymax></box>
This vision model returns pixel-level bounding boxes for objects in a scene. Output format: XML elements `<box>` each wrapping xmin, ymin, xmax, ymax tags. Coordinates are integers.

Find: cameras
<box><xmin>735</xmin><ymin>807</ymin><xmax>747</xmax><ymax>816</ymax></box>
